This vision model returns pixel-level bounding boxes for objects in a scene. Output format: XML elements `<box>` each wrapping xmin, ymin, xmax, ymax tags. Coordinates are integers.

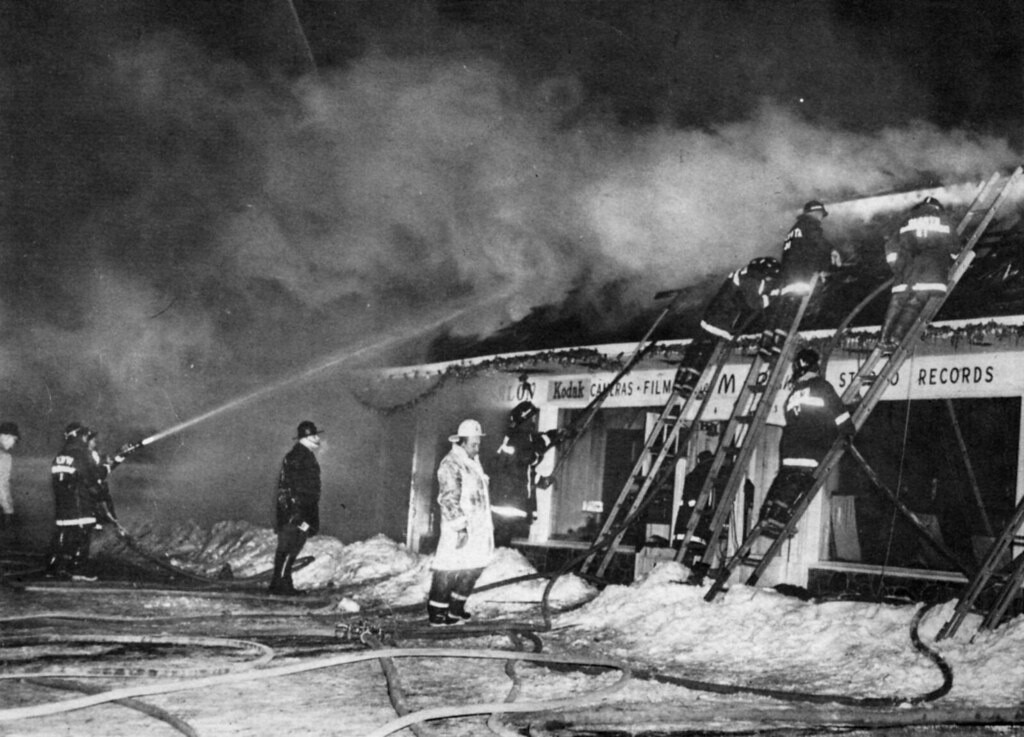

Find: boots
<box><xmin>427</xmin><ymin>597</ymin><xmax>459</xmax><ymax>626</ymax></box>
<box><xmin>770</xmin><ymin>329</ymin><xmax>786</xmax><ymax>356</ymax></box>
<box><xmin>46</xmin><ymin>549</ymin><xmax>68</xmax><ymax>577</ymax></box>
<box><xmin>70</xmin><ymin>557</ymin><xmax>99</xmax><ymax>583</ymax></box>
<box><xmin>672</xmin><ymin>367</ymin><xmax>705</xmax><ymax>401</ymax></box>
<box><xmin>758</xmin><ymin>332</ymin><xmax>770</xmax><ymax>357</ymax></box>
<box><xmin>760</xmin><ymin>499</ymin><xmax>797</xmax><ymax>535</ymax></box>
<box><xmin>447</xmin><ymin>590</ymin><xmax>472</xmax><ymax>621</ymax></box>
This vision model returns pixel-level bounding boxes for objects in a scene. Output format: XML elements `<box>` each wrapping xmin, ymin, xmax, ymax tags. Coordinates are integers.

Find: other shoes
<box><xmin>270</xmin><ymin>577</ymin><xmax>306</xmax><ymax>596</ymax></box>
<box><xmin>878</xmin><ymin>334</ymin><xmax>899</xmax><ymax>353</ymax></box>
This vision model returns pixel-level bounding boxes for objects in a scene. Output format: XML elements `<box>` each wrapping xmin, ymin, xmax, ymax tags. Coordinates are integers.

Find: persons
<box><xmin>427</xmin><ymin>419</ymin><xmax>495</xmax><ymax>627</ymax></box>
<box><xmin>0</xmin><ymin>423</ymin><xmax>23</xmax><ymax>581</ymax></box>
<box><xmin>489</xmin><ymin>401</ymin><xmax>568</xmax><ymax>548</ymax></box>
<box><xmin>877</xmin><ymin>197</ymin><xmax>963</xmax><ymax>355</ymax></box>
<box><xmin>758</xmin><ymin>200</ymin><xmax>834</xmax><ymax>355</ymax></box>
<box><xmin>672</xmin><ymin>256</ymin><xmax>779</xmax><ymax>391</ymax></box>
<box><xmin>673</xmin><ymin>451</ymin><xmax>715</xmax><ymax>546</ymax></box>
<box><xmin>269</xmin><ymin>421</ymin><xmax>325</xmax><ymax>596</ymax></box>
<box><xmin>760</xmin><ymin>349</ymin><xmax>856</xmax><ymax>533</ymax></box>
<box><xmin>39</xmin><ymin>423</ymin><xmax>117</xmax><ymax>582</ymax></box>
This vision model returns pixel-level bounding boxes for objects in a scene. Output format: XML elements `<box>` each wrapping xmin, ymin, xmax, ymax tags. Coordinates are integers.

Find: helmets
<box><xmin>291</xmin><ymin>420</ymin><xmax>323</xmax><ymax>441</ymax></box>
<box><xmin>791</xmin><ymin>350</ymin><xmax>819</xmax><ymax>379</ymax></box>
<box><xmin>64</xmin><ymin>422</ymin><xmax>86</xmax><ymax>442</ymax></box>
<box><xmin>448</xmin><ymin>420</ymin><xmax>488</xmax><ymax>444</ymax></box>
<box><xmin>764</xmin><ymin>257</ymin><xmax>782</xmax><ymax>278</ymax></box>
<box><xmin>803</xmin><ymin>201</ymin><xmax>829</xmax><ymax>218</ymax></box>
<box><xmin>509</xmin><ymin>401</ymin><xmax>539</xmax><ymax>424</ymax></box>
<box><xmin>0</xmin><ymin>423</ymin><xmax>20</xmax><ymax>439</ymax></box>
<box><xmin>923</xmin><ymin>197</ymin><xmax>946</xmax><ymax>214</ymax></box>
<box><xmin>84</xmin><ymin>428</ymin><xmax>97</xmax><ymax>440</ymax></box>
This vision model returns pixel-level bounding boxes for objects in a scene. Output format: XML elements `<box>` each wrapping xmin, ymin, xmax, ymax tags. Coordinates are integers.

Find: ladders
<box><xmin>676</xmin><ymin>273</ymin><xmax>818</xmax><ymax>577</ymax></box>
<box><xmin>704</xmin><ymin>163</ymin><xmax>1024</xmax><ymax>601</ymax></box>
<box><xmin>934</xmin><ymin>495</ymin><xmax>1024</xmax><ymax>647</ymax></box>
<box><xmin>581</xmin><ymin>311</ymin><xmax>742</xmax><ymax>586</ymax></box>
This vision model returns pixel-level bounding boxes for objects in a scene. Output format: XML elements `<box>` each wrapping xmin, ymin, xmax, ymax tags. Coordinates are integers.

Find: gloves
<box><xmin>538</xmin><ymin>476</ymin><xmax>558</xmax><ymax>491</ymax></box>
<box><xmin>556</xmin><ymin>428</ymin><xmax>577</xmax><ymax>440</ymax></box>
<box><xmin>830</xmin><ymin>249</ymin><xmax>844</xmax><ymax>269</ymax></box>
